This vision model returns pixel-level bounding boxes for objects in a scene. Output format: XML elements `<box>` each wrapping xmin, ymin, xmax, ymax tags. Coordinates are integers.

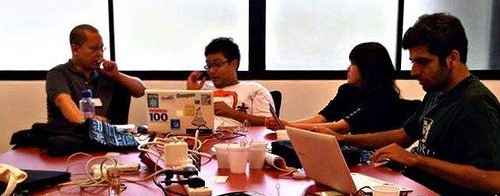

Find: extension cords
<box><xmin>90</xmin><ymin>163</ymin><xmax>140</xmax><ymax>172</ymax></box>
<box><xmin>173</xmin><ymin>160</ymin><xmax>213</xmax><ymax>196</ymax></box>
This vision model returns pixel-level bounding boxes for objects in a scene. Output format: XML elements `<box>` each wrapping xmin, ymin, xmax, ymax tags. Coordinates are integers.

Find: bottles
<box><xmin>79</xmin><ymin>89</ymin><xmax>95</xmax><ymax>116</ymax></box>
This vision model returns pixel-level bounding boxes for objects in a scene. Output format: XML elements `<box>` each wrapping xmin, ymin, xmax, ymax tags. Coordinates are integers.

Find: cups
<box><xmin>214</xmin><ymin>143</ymin><xmax>229</xmax><ymax>169</ymax></box>
<box><xmin>227</xmin><ymin>144</ymin><xmax>250</xmax><ymax>174</ymax></box>
<box><xmin>276</xmin><ymin>130</ymin><xmax>289</xmax><ymax>140</ymax></box>
<box><xmin>247</xmin><ymin>142</ymin><xmax>268</xmax><ymax>169</ymax></box>
<box><xmin>371</xmin><ymin>185</ymin><xmax>401</xmax><ymax>196</ymax></box>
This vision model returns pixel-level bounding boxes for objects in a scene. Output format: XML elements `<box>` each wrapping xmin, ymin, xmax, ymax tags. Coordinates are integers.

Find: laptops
<box><xmin>145</xmin><ymin>89</ymin><xmax>215</xmax><ymax>135</ymax></box>
<box><xmin>269</xmin><ymin>103</ymin><xmax>415</xmax><ymax>195</ymax></box>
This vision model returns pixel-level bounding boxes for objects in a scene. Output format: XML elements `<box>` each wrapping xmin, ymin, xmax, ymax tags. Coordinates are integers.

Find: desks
<box><xmin>0</xmin><ymin>127</ymin><xmax>442</xmax><ymax>196</ymax></box>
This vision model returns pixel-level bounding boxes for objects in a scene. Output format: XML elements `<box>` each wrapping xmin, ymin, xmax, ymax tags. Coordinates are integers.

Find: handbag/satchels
<box><xmin>271</xmin><ymin>139</ymin><xmax>364</xmax><ymax>168</ymax></box>
<box><xmin>0</xmin><ymin>163</ymin><xmax>71</xmax><ymax>196</ymax></box>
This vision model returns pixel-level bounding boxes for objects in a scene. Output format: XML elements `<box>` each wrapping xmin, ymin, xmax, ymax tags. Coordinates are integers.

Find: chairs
<box><xmin>104</xmin><ymin>90</ymin><xmax>131</xmax><ymax>124</ymax></box>
<box><xmin>399</xmin><ymin>97</ymin><xmax>421</xmax><ymax>128</ymax></box>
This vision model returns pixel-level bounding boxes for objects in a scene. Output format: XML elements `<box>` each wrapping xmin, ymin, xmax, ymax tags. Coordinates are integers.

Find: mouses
<box><xmin>137</xmin><ymin>124</ymin><xmax>148</xmax><ymax>134</ymax></box>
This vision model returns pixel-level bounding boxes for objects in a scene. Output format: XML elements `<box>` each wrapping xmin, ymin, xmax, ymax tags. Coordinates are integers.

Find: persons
<box><xmin>314</xmin><ymin>12</ymin><xmax>500</xmax><ymax>196</ymax></box>
<box><xmin>46</xmin><ymin>24</ymin><xmax>146</xmax><ymax>128</ymax></box>
<box><xmin>265</xmin><ymin>42</ymin><xmax>398</xmax><ymax>151</ymax></box>
<box><xmin>186</xmin><ymin>37</ymin><xmax>276</xmax><ymax>127</ymax></box>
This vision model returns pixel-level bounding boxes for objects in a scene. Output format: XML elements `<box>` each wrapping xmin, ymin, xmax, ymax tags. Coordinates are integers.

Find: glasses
<box><xmin>204</xmin><ymin>60</ymin><xmax>228</xmax><ymax>69</ymax></box>
<box><xmin>87</xmin><ymin>45</ymin><xmax>106</xmax><ymax>52</ymax></box>
